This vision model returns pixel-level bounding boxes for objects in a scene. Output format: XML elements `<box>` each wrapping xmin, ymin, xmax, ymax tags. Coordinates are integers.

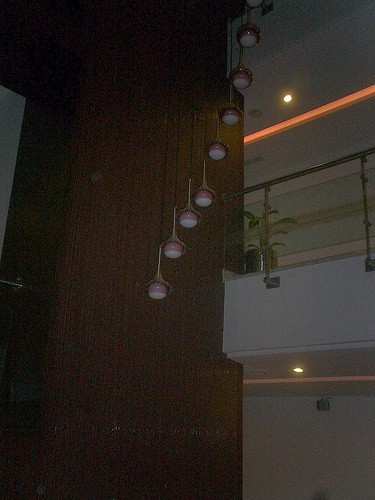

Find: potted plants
<box><xmin>240</xmin><ymin>209</ymin><xmax>300</xmax><ymax>273</ymax></box>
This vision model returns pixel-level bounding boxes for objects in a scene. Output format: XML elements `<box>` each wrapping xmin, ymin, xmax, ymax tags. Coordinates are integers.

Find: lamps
<box><xmin>220</xmin><ymin>20</ymin><xmax>243</xmax><ymax>126</ymax></box>
<box><xmin>230</xmin><ymin>15</ymin><xmax>255</xmax><ymax>90</ymax></box>
<box><xmin>163</xmin><ymin>208</ymin><xmax>185</xmax><ymax>259</ymax></box>
<box><xmin>178</xmin><ymin>178</ymin><xmax>202</xmax><ymax>228</ymax></box>
<box><xmin>236</xmin><ymin>3</ymin><xmax>260</xmax><ymax>48</ymax></box>
<box><xmin>208</xmin><ymin>114</ymin><xmax>229</xmax><ymax>161</ymax></box>
<box><xmin>144</xmin><ymin>244</ymin><xmax>172</xmax><ymax>300</ymax></box>
<box><xmin>245</xmin><ymin>1</ymin><xmax>263</xmax><ymax>8</ymax></box>
<box><xmin>194</xmin><ymin>159</ymin><xmax>217</xmax><ymax>207</ymax></box>
